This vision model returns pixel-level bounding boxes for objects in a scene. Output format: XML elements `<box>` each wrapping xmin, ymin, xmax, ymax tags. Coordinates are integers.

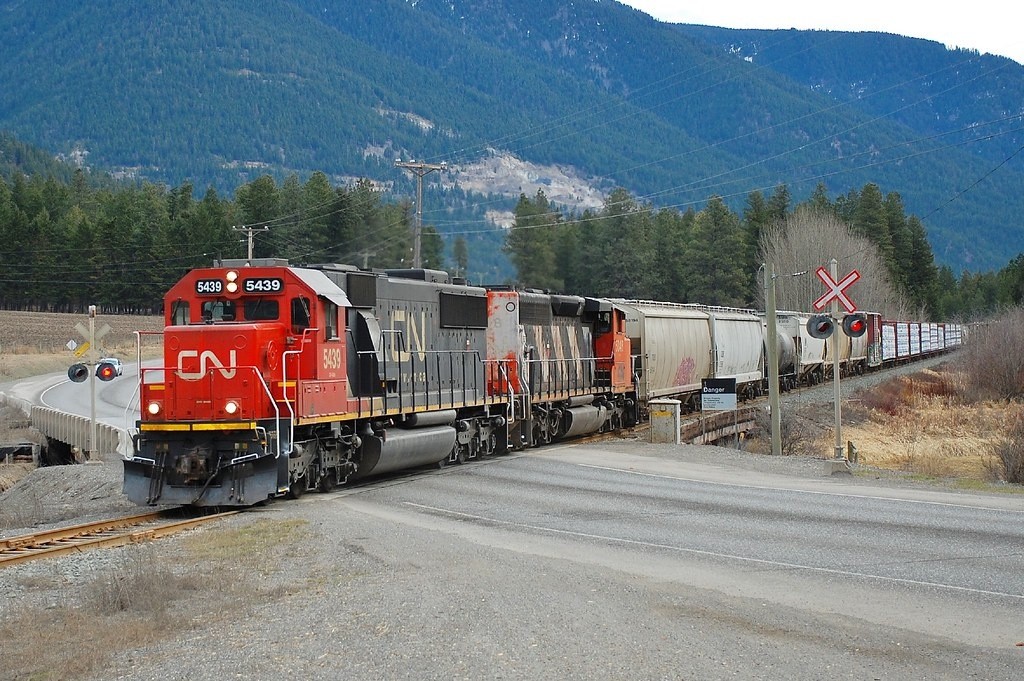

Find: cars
<box><xmin>95</xmin><ymin>358</ymin><xmax>123</xmax><ymax>377</ymax></box>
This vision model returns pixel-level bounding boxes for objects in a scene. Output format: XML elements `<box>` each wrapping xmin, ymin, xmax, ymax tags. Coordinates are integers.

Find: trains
<box><xmin>122</xmin><ymin>258</ymin><xmax>985</xmax><ymax>507</ymax></box>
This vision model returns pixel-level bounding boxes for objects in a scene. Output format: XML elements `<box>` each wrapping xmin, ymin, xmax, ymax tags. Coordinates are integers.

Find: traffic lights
<box><xmin>844</xmin><ymin>314</ymin><xmax>866</xmax><ymax>338</ymax></box>
<box><xmin>96</xmin><ymin>363</ymin><xmax>116</xmax><ymax>382</ymax></box>
<box><xmin>68</xmin><ymin>364</ymin><xmax>88</xmax><ymax>383</ymax></box>
<box><xmin>810</xmin><ymin>316</ymin><xmax>834</xmax><ymax>339</ymax></box>
